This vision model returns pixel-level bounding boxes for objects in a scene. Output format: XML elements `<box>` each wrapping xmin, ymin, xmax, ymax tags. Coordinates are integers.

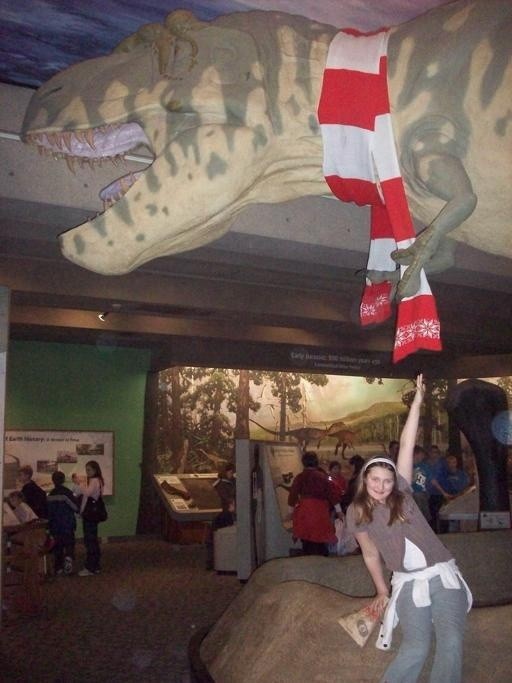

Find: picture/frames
<box><xmin>3</xmin><ymin>427</ymin><xmax>117</xmax><ymax>499</ymax></box>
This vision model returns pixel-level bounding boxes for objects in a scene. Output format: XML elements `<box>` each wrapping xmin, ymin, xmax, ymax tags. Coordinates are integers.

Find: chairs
<box><xmin>3</xmin><ymin>517</ymin><xmax>55</xmax><ymax>590</ymax></box>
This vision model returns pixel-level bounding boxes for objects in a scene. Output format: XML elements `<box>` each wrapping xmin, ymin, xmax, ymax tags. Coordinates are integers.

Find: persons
<box><xmin>347</xmin><ymin>370</ymin><xmax>473</xmax><ymax>683</ymax></box>
<box><xmin>1</xmin><ymin>459</ymin><xmax>109</xmax><ymax>583</ymax></box>
<box><xmin>205</xmin><ymin>437</ymin><xmax>475</xmax><ymax>569</ymax></box>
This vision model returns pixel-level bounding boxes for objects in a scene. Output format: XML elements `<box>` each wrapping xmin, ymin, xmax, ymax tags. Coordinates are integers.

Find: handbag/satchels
<box><xmin>84</xmin><ymin>496</ymin><xmax>107</xmax><ymax>523</ymax></box>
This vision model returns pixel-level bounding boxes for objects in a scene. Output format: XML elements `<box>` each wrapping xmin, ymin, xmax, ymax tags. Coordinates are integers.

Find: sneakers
<box><xmin>64</xmin><ymin>556</ymin><xmax>72</xmax><ymax>574</ymax></box>
<box><xmin>78</xmin><ymin>570</ymin><xmax>93</xmax><ymax>576</ymax></box>
